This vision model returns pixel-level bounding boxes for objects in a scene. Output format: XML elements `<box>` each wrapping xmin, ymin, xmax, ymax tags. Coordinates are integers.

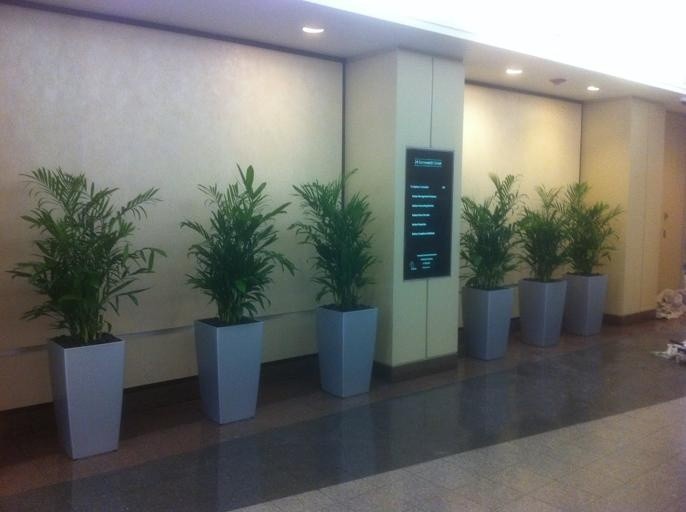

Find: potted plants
<box><xmin>459</xmin><ymin>168</ymin><xmax>529</xmax><ymax>364</ymax></box>
<box><xmin>284</xmin><ymin>165</ymin><xmax>382</xmax><ymax>400</ymax></box>
<box><xmin>3</xmin><ymin>164</ymin><xmax>168</xmax><ymax>462</ymax></box>
<box><xmin>555</xmin><ymin>180</ymin><xmax>626</xmax><ymax>340</ymax></box>
<box><xmin>177</xmin><ymin>161</ymin><xmax>300</xmax><ymax>427</ymax></box>
<box><xmin>516</xmin><ymin>178</ymin><xmax>582</xmax><ymax>350</ymax></box>
